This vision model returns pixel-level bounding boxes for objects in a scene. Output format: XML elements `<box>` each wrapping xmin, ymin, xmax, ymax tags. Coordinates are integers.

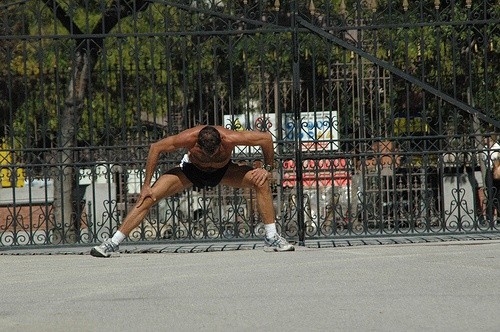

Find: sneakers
<box><xmin>262</xmin><ymin>234</ymin><xmax>295</xmax><ymax>253</ymax></box>
<box><xmin>90</xmin><ymin>239</ymin><xmax>122</xmax><ymax>259</ymax></box>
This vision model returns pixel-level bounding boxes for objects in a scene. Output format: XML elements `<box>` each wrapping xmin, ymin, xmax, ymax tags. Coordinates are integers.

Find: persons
<box><xmin>475</xmin><ymin>133</ymin><xmax>500</xmax><ymax>228</ymax></box>
<box><xmin>90</xmin><ymin>124</ymin><xmax>296</xmax><ymax>258</ymax></box>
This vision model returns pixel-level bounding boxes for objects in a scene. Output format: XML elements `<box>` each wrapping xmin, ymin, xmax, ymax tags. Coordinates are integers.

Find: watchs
<box><xmin>262</xmin><ymin>163</ymin><xmax>274</xmax><ymax>172</ymax></box>
